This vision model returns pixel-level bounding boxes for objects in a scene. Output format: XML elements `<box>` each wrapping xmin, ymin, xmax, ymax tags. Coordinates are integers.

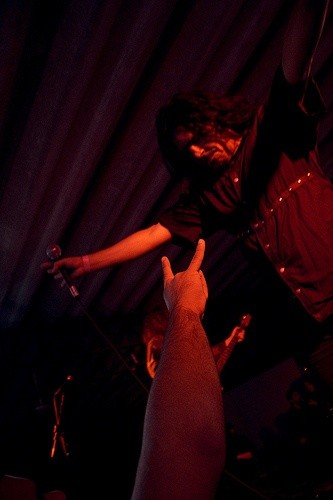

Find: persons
<box><xmin>39</xmin><ymin>0</ymin><xmax>333</xmax><ymax>337</ymax></box>
<box><xmin>2</xmin><ymin>235</ymin><xmax>333</xmax><ymax>499</ymax></box>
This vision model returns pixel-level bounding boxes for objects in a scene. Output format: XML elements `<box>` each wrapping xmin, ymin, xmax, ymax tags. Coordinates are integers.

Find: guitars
<box><xmin>214</xmin><ymin>314</ymin><xmax>253</xmax><ymax>392</ymax></box>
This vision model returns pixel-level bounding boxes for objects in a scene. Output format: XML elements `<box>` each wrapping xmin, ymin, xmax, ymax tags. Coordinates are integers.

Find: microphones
<box><xmin>55</xmin><ymin>375</ymin><xmax>73</xmax><ymax>395</ymax></box>
<box><xmin>46</xmin><ymin>243</ymin><xmax>79</xmax><ymax>298</ymax></box>
